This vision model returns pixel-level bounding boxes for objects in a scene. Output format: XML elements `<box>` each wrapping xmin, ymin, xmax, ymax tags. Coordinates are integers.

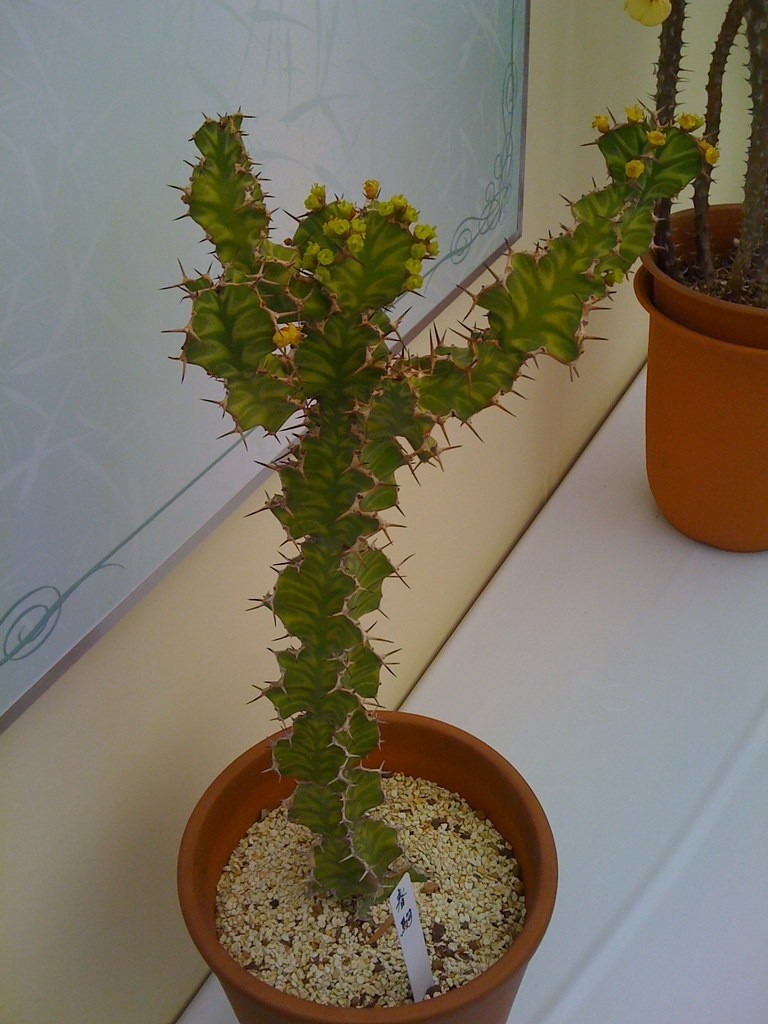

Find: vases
<box><xmin>177</xmin><ymin>708</ymin><xmax>558</xmax><ymax>1024</ymax></box>
<box><xmin>633</xmin><ymin>262</ymin><xmax>768</xmax><ymax>554</ymax></box>
<box><xmin>640</xmin><ymin>203</ymin><xmax>767</xmax><ymax>351</ymax></box>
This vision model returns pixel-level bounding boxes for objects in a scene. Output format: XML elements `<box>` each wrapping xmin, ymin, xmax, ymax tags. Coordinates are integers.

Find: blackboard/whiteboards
<box><xmin>0</xmin><ymin>1</ymin><xmax>528</xmax><ymax>732</ymax></box>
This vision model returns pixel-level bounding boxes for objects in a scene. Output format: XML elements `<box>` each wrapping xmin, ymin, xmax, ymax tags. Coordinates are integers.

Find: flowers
<box><xmin>624</xmin><ymin>0</ymin><xmax>768</xmax><ymax>307</ymax></box>
<box><xmin>159</xmin><ymin>102</ymin><xmax>723</xmax><ymax>909</ymax></box>
